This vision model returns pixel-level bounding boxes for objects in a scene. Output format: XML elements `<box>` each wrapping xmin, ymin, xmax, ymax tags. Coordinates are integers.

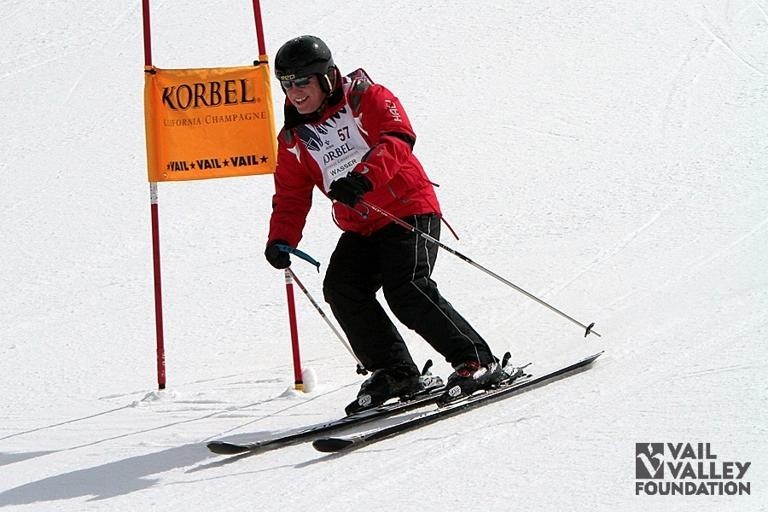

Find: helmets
<box><xmin>274</xmin><ymin>35</ymin><xmax>337</xmax><ymax>95</ymax></box>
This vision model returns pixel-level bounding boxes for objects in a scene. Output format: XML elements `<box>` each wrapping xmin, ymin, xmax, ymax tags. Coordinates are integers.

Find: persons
<box><xmin>265</xmin><ymin>35</ymin><xmax>522</xmax><ymax>414</ymax></box>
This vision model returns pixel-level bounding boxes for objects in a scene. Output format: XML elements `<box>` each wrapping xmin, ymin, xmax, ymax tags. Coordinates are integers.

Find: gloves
<box><xmin>327</xmin><ymin>171</ymin><xmax>373</xmax><ymax>208</ymax></box>
<box><xmin>264</xmin><ymin>240</ymin><xmax>292</xmax><ymax>270</ymax></box>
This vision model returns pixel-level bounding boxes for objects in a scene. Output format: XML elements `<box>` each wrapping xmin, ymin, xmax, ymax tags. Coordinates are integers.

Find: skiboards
<box><xmin>207</xmin><ymin>350</ymin><xmax>603</xmax><ymax>454</ymax></box>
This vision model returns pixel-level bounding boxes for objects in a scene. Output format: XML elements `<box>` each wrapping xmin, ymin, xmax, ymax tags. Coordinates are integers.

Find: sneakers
<box><xmin>434</xmin><ymin>356</ymin><xmax>502</xmax><ymax>408</ymax></box>
<box><xmin>356</xmin><ymin>360</ymin><xmax>421</xmax><ymax>408</ymax></box>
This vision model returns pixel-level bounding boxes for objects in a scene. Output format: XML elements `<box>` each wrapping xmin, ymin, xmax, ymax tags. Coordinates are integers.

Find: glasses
<box><xmin>280</xmin><ymin>76</ymin><xmax>313</xmax><ymax>89</ymax></box>
